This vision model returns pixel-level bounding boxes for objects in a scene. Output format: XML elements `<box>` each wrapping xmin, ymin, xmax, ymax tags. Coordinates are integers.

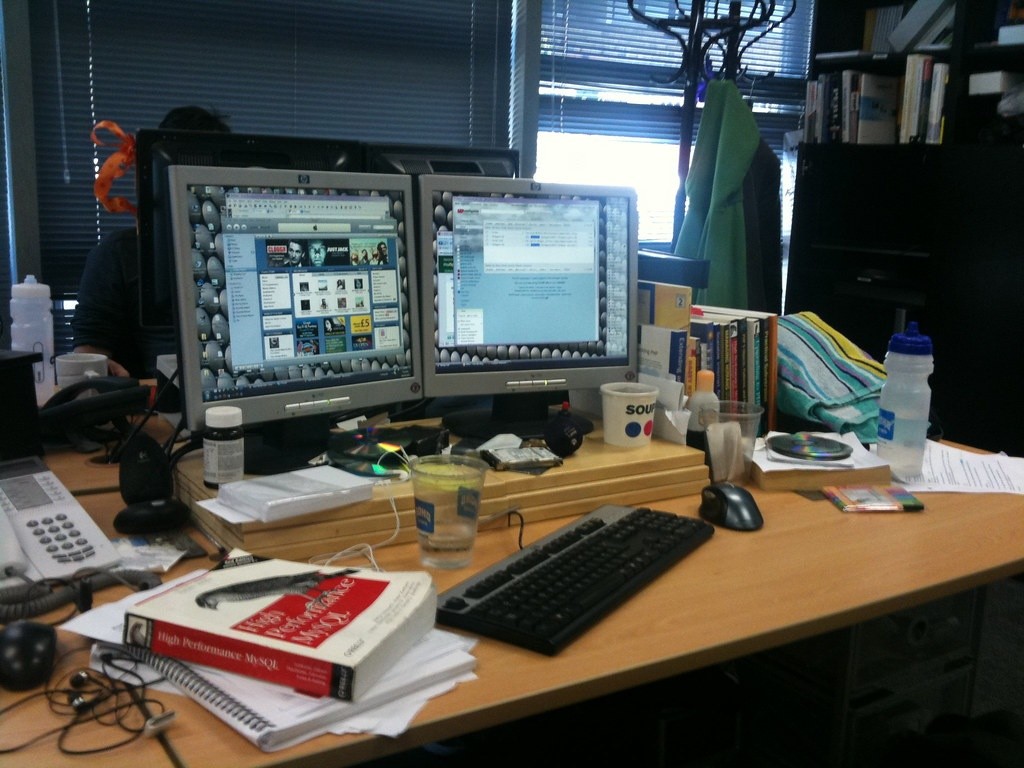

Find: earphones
<box><xmin>70</xmin><ymin>671</ymin><xmax>102</xmax><ymax>689</ymax></box>
<box><xmin>67</xmin><ymin>691</ymin><xmax>100</xmax><ymax>714</ymax></box>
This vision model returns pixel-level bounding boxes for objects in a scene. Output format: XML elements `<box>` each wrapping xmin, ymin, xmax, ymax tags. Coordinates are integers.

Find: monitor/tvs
<box><xmin>362</xmin><ymin>142</ymin><xmax>520</xmax><ymax>177</ymax></box>
<box><xmin>135</xmin><ymin>127</ymin><xmax>362</xmax><ymax>330</ymax></box>
<box><xmin>167</xmin><ymin>163</ymin><xmax>422</xmax><ymax>474</ymax></box>
<box><xmin>418</xmin><ymin>174</ymin><xmax>638</xmax><ymax>439</ymax></box>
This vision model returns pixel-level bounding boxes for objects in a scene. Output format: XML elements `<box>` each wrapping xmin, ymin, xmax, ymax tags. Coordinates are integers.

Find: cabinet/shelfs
<box><xmin>785</xmin><ymin>0</ymin><xmax>1024</xmax><ymax>456</ymax></box>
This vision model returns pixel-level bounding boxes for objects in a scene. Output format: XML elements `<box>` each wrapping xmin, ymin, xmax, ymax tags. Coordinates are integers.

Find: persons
<box><xmin>71</xmin><ymin>105</ymin><xmax>235</xmax><ymax>413</ymax></box>
<box><xmin>283</xmin><ymin>239</ymin><xmax>389</xmax><ymax>358</ymax></box>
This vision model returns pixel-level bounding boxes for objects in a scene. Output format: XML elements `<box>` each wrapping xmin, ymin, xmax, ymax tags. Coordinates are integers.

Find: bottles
<box><xmin>685</xmin><ymin>370</ymin><xmax>720</xmax><ymax>479</ymax></box>
<box><xmin>10</xmin><ymin>275</ymin><xmax>55</xmax><ymax>406</ymax></box>
<box><xmin>203</xmin><ymin>406</ymin><xmax>245</xmax><ymax>491</ymax></box>
<box><xmin>874</xmin><ymin>322</ymin><xmax>933</xmax><ymax>479</ymax></box>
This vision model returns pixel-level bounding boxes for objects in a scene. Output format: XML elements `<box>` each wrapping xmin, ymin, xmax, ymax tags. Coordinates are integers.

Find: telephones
<box><xmin>38</xmin><ymin>376</ymin><xmax>153</xmax><ymax>427</ymax></box>
<box><xmin>1</xmin><ymin>453</ymin><xmax>121</xmax><ymax>596</ymax></box>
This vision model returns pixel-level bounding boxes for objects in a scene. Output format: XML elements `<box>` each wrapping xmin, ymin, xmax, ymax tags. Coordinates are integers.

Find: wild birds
<box><xmin>195</xmin><ymin>567</ymin><xmax>360</xmax><ymax>611</ymax></box>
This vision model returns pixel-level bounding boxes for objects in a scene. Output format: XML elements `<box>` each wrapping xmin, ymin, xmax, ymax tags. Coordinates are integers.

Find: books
<box><xmin>634</xmin><ymin>276</ymin><xmax>780</xmax><ymax>436</ymax></box>
<box><xmin>752</xmin><ymin>431</ymin><xmax>893</xmax><ymax>492</ymax></box>
<box><xmin>120</xmin><ymin>547</ymin><xmax>481</xmax><ymax>754</ymax></box>
<box><xmin>804</xmin><ymin>0</ymin><xmax>956</xmax><ymax>144</ymax></box>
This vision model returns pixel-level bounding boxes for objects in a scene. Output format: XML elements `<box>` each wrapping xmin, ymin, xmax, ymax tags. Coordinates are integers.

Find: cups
<box><xmin>55</xmin><ymin>353</ymin><xmax>108</xmax><ymax>398</ymax></box>
<box><xmin>410</xmin><ymin>455</ymin><xmax>487</xmax><ymax>568</ymax></box>
<box><xmin>600</xmin><ymin>381</ymin><xmax>659</xmax><ymax>448</ymax></box>
<box><xmin>702</xmin><ymin>400</ymin><xmax>765</xmax><ymax>487</ymax></box>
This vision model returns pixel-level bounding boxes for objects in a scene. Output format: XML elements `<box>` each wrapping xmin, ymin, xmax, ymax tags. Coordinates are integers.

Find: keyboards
<box><xmin>435</xmin><ymin>502</ymin><xmax>714</xmax><ymax>657</ymax></box>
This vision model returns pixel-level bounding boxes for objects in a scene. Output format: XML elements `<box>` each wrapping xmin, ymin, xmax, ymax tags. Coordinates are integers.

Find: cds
<box><xmin>767</xmin><ymin>434</ymin><xmax>853</xmax><ymax>461</ymax></box>
<box><xmin>327</xmin><ymin>428</ymin><xmax>411</xmax><ymax>477</ymax></box>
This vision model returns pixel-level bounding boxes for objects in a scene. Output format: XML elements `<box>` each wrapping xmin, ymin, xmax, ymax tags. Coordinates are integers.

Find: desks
<box><xmin>0</xmin><ymin>413</ymin><xmax>1024</xmax><ymax>768</ymax></box>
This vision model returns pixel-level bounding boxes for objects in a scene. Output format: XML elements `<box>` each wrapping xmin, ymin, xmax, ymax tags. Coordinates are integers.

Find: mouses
<box><xmin>0</xmin><ymin>619</ymin><xmax>56</xmax><ymax>691</ymax></box>
<box><xmin>699</xmin><ymin>483</ymin><xmax>764</xmax><ymax>530</ymax></box>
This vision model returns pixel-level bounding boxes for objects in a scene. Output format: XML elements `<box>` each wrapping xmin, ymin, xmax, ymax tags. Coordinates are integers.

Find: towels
<box><xmin>775</xmin><ymin>310</ymin><xmax>888</xmax><ymax>446</ymax></box>
